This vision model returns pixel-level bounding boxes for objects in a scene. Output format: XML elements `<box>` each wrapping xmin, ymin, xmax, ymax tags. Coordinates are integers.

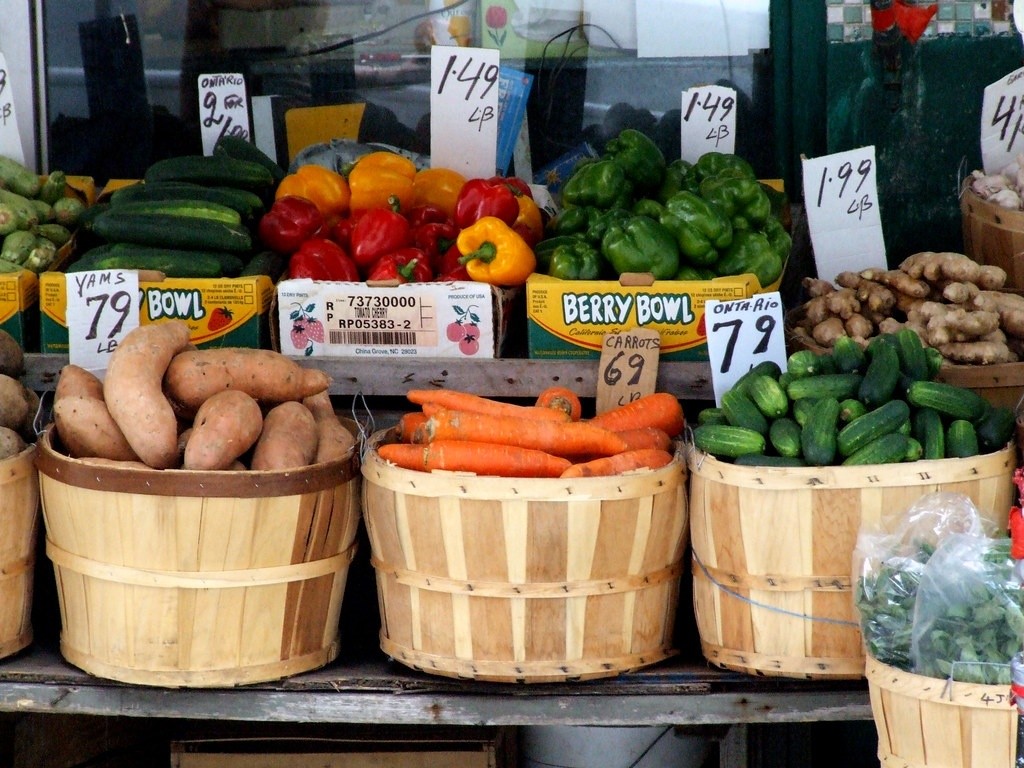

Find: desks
<box><xmin>0</xmin><ymin>636</ymin><xmax>868</xmax><ymax>728</ymax></box>
<box><xmin>9</xmin><ymin>319</ymin><xmax>770</xmax><ymax>413</ymax></box>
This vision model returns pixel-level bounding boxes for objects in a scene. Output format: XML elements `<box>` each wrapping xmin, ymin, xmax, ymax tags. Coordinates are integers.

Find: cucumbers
<box><xmin>68</xmin><ymin>134</ymin><xmax>286</xmax><ymax>284</ymax></box>
<box><xmin>693</xmin><ymin>330</ymin><xmax>1014</xmax><ymax>466</ymax></box>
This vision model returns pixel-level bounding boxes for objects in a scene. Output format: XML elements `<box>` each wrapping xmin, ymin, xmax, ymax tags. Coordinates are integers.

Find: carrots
<box><xmin>378</xmin><ymin>386</ymin><xmax>682</xmax><ymax>478</ymax></box>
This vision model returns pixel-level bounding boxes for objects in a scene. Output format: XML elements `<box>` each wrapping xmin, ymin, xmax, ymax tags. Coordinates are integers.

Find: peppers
<box><xmin>261</xmin><ymin>128</ymin><xmax>792</xmax><ymax>289</ymax></box>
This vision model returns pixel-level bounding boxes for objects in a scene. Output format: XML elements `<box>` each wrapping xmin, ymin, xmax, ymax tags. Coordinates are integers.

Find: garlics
<box><xmin>972</xmin><ymin>150</ymin><xmax>1024</xmax><ymax>210</ymax></box>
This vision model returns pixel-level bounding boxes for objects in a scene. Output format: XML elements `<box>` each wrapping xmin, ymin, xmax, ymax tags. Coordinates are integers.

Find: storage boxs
<box><xmin>678</xmin><ymin>436</ymin><xmax>1015</xmax><ymax>678</ymax></box>
<box><xmin>41</xmin><ymin>180</ymin><xmax>286</xmax><ymax>363</ymax></box>
<box><xmin>1</xmin><ymin>440</ymin><xmax>44</xmax><ymax>663</ymax></box>
<box><xmin>848</xmin><ymin>646</ymin><xmax>1020</xmax><ymax>765</ymax></box>
<box><xmin>275</xmin><ymin>185</ymin><xmax>549</xmax><ymax>358</ymax></box>
<box><xmin>358</xmin><ymin>426</ymin><xmax>683</xmax><ymax>687</ymax></box>
<box><xmin>794</xmin><ymin>322</ymin><xmax>1024</xmax><ymax>437</ymax></box>
<box><xmin>525</xmin><ymin>176</ymin><xmax>789</xmax><ymax>356</ymax></box>
<box><xmin>961</xmin><ymin>172</ymin><xmax>1023</xmax><ymax>297</ymax></box>
<box><xmin>31</xmin><ymin>420</ymin><xmax>360</xmax><ymax>690</ymax></box>
<box><xmin>0</xmin><ymin>173</ymin><xmax>91</xmax><ymax>351</ymax></box>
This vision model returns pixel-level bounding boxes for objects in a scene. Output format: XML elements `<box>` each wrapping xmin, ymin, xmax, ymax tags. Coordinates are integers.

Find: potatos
<box><xmin>0</xmin><ymin>320</ymin><xmax>357</xmax><ymax>476</ymax></box>
